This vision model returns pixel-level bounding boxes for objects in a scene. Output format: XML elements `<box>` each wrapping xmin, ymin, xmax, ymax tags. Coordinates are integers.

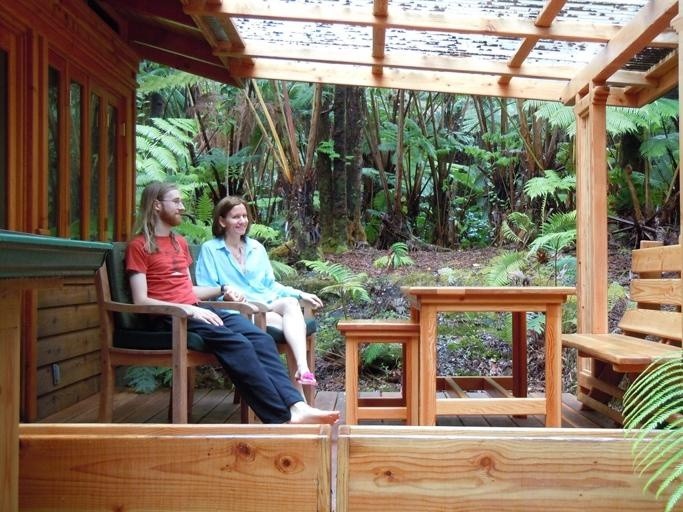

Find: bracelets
<box><xmin>220</xmin><ymin>282</ymin><xmax>226</xmax><ymax>296</ymax></box>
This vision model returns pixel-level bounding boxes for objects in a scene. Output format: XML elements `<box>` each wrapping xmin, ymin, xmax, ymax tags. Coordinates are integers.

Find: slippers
<box><xmin>295</xmin><ymin>372</ymin><xmax>318</xmax><ymax>387</ymax></box>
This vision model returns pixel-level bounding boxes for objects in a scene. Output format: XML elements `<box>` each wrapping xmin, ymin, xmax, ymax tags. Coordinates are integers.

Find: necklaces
<box><xmin>228</xmin><ymin>242</ymin><xmax>243</xmax><ymax>263</ymax></box>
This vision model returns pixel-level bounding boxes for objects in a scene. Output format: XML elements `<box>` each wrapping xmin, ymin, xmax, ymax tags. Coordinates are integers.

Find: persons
<box><xmin>195</xmin><ymin>196</ymin><xmax>325</xmax><ymax>404</ymax></box>
<box><xmin>126</xmin><ymin>180</ymin><xmax>340</xmax><ymax>425</ymax></box>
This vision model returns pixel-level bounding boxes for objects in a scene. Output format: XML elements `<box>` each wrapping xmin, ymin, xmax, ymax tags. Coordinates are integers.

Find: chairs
<box><xmin>561</xmin><ymin>239</ymin><xmax>683</xmax><ymax>430</ymax></box>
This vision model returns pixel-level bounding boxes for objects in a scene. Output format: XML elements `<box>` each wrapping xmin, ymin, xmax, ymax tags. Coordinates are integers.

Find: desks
<box><xmin>335</xmin><ymin>317</ymin><xmax>419</xmax><ymax>426</ymax></box>
<box><xmin>398</xmin><ymin>285</ymin><xmax>576</xmax><ymax>427</ymax></box>
